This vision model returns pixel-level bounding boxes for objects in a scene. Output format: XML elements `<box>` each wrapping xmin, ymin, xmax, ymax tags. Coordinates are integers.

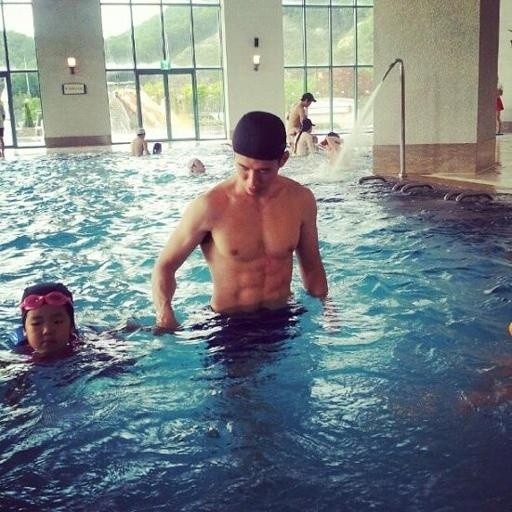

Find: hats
<box><xmin>303</xmin><ymin>93</ymin><xmax>316</xmax><ymax>102</ymax></box>
<box><xmin>232</xmin><ymin>112</ymin><xmax>286</xmax><ymax>160</ymax></box>
<box><xmin>302</xmin><ymin>118</ymin><xmax>315</xmax><ymax>127</ymax></box>
<box><xmin>136</xmin><ymin>128</ymin><xmax>145</xmax><ymax>135</ymax></box>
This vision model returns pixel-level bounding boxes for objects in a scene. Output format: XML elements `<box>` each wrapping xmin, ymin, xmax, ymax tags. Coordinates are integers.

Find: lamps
<box><xmin>65</xmin><ymin>57</ymin><xmax>78</xmax><ymax>74</ymax></box>
<box><xmin>252</xmin><ymin>54</ymin><xmax>262</xmax><ymax>72</ymax></box>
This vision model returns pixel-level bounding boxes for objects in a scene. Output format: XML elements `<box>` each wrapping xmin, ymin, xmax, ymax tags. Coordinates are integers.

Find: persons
<box><xmin>14</xmin><ymin>281</ymin><xmax>78</xmax><ymax>366</ymax></box>
<box><xmin>131</xmin><ymin>128</ymin><xmax>150</xmax><ymax>157</ymax></box>
<box><xmin>152</xmin><ymin>112</ymin><xmax>329</xmax><ymax>349</ymax></box>
<box><xmin>153</xmin><ymin>142</ymin><xmax>162</xmax><ymax>155</ymax></box>
<box><xmin>326</xmin><ymin>132</ymin><xmax>341</xmax><ymax>157</ymax></box>
<box><xmin>496</xmin><ymin>84</ymin><xmax>504</xmax><ymax>135</ymax></box>
<box><xmin>455</xmin><ymin>318</ymin><xmax>512</xmax><ymax>411</ymax></box>
<box><xmin>188</xmin><ymin>158</ymin><xmax>206</xmax><ymax>174</ymax></box>
<box><xmin>287</xmin><ymin>92</ymin><xmax>317</xmax><ymax>143</ymax></box>
<box><xmin>0</xmin><ymin>99</ymin><xmax>5</xmax><ymax>160</ymax></box>
<box><xmin>292</xmin><ymin>118</ymin><xmax>317</xmax><ymax>155</ymax></box>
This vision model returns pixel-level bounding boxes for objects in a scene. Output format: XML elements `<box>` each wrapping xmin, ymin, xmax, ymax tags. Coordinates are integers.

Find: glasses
<box><xmin>19</xmin><ymin>291</ymin><xmax>74</xmax><ymax>310</ymax></box>
